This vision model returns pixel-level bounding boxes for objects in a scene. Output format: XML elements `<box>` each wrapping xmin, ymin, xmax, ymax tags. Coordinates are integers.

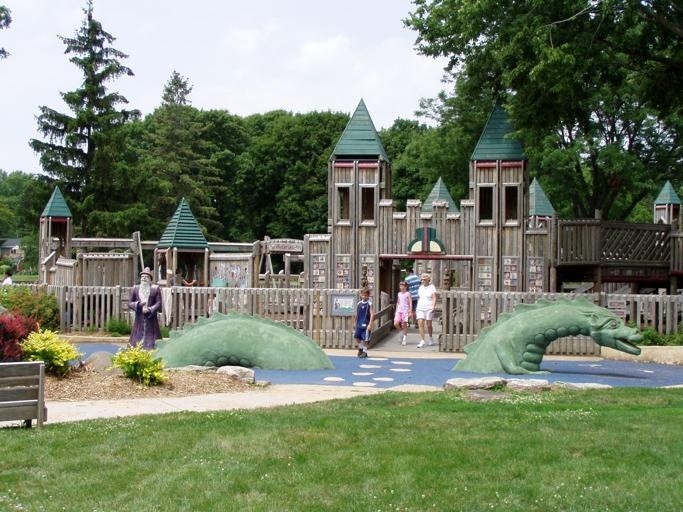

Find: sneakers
<box><xmin>359</xmin><ymin>352</ymin><xmax>366</xmax><ymax>358</ymax></box>
<box><xmin>427</xmin><ymin>338</ymin><xmax>433</xmax><ymax>346</ymax></box>
<box><xmin>416</xmin><ymin>343</ymin><xmax>425</xmax><ymax>349</ymax></box>
<box><xmin>357</xmin><ymin>348</ymin><xmax>363</xmax><ymax>356</ymax></box>
<box><xmin>401</xmin><ymin>339</ymin><xmax>406</xmax><ymax>346</ymax></box>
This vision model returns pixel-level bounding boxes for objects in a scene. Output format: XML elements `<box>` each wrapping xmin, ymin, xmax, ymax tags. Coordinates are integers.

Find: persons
<box><xmin>128</xmin><ymin>267</ymin><xmax>162</xmax><ymax>352</ymax></box>
<box><xmin>170</xmin><ymin>267</ymin><xmax>197</xmax><ymax>329</ymax></box>
<box><xmin>0</xmin><ymin>272</ymin><xmax>13</xmax><ymax>296</ymax></box>
<box><xmin>353</xmin><ymin>289</ymin><xmax>375</xmax><ymax>358</ymax></box>
<box><xmin>394</xmin><ymin>270</ymin><xmax>437</xmax><ymax>348</ymax></box>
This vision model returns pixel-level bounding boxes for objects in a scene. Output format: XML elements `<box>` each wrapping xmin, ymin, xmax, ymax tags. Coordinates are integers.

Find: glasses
<box><xmin>420</xmin><ymin>279</ymin><xmax>429</xmax><ymax>281</ymax></box>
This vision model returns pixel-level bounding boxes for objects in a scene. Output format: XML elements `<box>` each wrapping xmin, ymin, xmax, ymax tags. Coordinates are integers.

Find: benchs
<box><xmin>0</xmin><ymin>359</ymin><xmax>49</xmax><ymax>433</ymax></box>
